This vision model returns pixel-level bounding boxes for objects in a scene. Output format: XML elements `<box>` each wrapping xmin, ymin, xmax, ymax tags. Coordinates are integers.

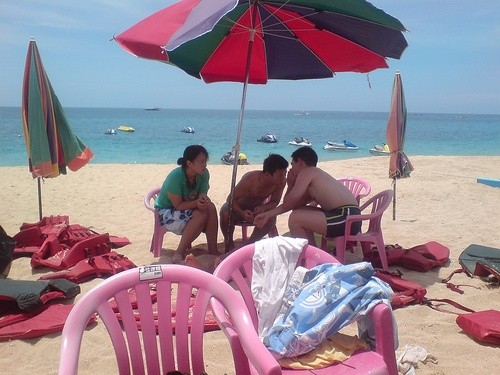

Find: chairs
<box><xmin>56</xmin><ymin>264</ymin><xmax>283</xmax><ymax>375</ymax></box>
<box><xmin>309</xmin><ymin>178</ymin><xmax>394</xmax><ymax>272</ymax></box>
<box><xmin>211</xmin><ymin>241</ymin><xmax>398</xmax><ymax>375</ymax></box>
<box><xmin>237</xmin><ymin>194</ymin><xmax>279</xmax><ymax>245</ymax></box>
<box><xmin>144</xmin><ymin>188</ymin><xmax>191</xmax><ymax>258</ymax></box>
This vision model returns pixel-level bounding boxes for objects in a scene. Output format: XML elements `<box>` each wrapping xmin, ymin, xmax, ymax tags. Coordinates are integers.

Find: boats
<box><xmin>180</xmin><ymin>126</ymin><xmax>196</xmax><ymax>134</ymax></box>
<box><xmin>105</xmin><ymin>128</ymin><xmax>118</xmax><ymax>134</ymax></box>
<box><xmin>370</xmin><ymin>143</ymin><xmax>391</xmax><ymax>156</ymax></box>
<box><xmin>288</xmin><ymin>137</ymin><xmax>313</xmax><ymax>147</ymax></box>
<box><xmin>118</xmin><ymin>126</ymin><xmax>135</xmax><ymax>132</ymax></box>
<box><xmin>220</xmin><ymin>152</ymin><xmax>247</xmax><ymax>165</ymax></box>
<box><xmin>323</xmin><ymin>139</ymin><xmax>358</xmax><ymax>151</ymax></box>
<box><xmin>257</xmin><ymin>133</ymin><xmax>278</xmax><ymax>143</ymax></box>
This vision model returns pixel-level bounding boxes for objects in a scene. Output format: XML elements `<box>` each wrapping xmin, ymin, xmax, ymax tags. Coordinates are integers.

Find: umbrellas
<box><xmin>22</xmin><ymin>36</ymin><xmax>95</xmax><ymax>221</ymax></box>
<box><xmin>386</xmin><ymin>71</ymin><xmax>414</xmax><ymax>220</ymax></box>
<box><xmin>109</xmin><ymin>0</ymin><xmax>408</xmax><ymax>252</ymax></box>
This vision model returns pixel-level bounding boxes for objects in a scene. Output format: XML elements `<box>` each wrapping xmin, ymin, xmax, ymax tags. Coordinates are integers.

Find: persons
<box><xmin>185</xmin><ymin>253</ymin><xmax>246</xmax><ymax>282</ymax></box>
<box><xmin>219</xmin><ymin>154</ymin><xmax>289</xmax><ymax>253</ymax></box>
<box><xmin>253</xmin><ymin>145</ymin><xmax>362</xmax><ymax>248</ymax></box>
<box><xmin>154</xmin><ymin>145</ymin><xmax>223</xmax><ymax>263</ymax></box>
